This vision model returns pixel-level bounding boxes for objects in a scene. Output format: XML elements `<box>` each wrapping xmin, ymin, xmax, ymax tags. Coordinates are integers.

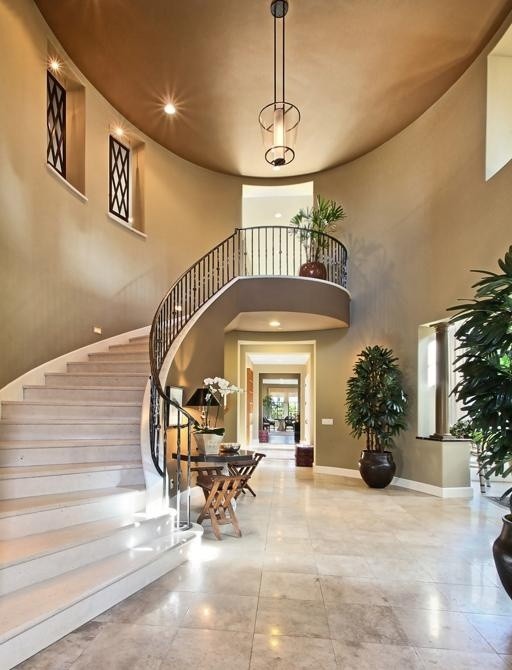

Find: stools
<box><xmin>293</xmin><ymin>443</ymin><xmax>315</xmax><ymax>468</ymax></box>
<box><xmin>259</xmin><ymin>429</ymin><xmax>270</xmax><ymax>443</ymax></box>
<box><xmin>262</xmin><ymin>423</ymin><xmax>271</xmax><ymax>433</ymax></box>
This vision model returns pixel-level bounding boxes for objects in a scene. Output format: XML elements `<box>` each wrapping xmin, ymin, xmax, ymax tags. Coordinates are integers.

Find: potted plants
<box><xmin>444</xmin><ymin>243</ymin><xmax>512</xmax><ymax>601</ymax></box>
<box><xmin>286</xmin><ymin>194</ymin><xmax>349</xmax><ymax>281</ymax></box>
<box><xmin>342</xmin><ymin>345</ymin><xmax>408</xmax><ymax>489</ymax></box>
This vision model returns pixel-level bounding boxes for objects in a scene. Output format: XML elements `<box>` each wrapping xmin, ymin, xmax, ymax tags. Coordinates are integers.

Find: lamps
<box><xmin>255</xmin><ymin>0</ymin><xmax>303</xmax><ymax>170</ymax></box>
<box><xmin>187</xmin><ymin>387</ymin><xmax>221</xmax><ymax>429</ymax></box>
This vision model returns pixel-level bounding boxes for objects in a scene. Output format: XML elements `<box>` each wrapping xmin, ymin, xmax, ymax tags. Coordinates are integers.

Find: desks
<box><xmin>275</xmin><ymin>418</ymin><xmax>292</xmax><ymax>433</ymax></box>
<box><xmin>171</xmin><ymin>450</ymin><xmax>254</xmax><ymax>522</ymax></box>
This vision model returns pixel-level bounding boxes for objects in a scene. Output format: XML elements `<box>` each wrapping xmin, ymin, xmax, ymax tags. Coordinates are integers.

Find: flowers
<box><xmin>191</xmin><ymin>376</ymin><xmax>242</xmax><ymax>436</ymax></box>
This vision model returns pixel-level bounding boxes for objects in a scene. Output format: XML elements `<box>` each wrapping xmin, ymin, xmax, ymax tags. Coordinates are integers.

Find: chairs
<box><xmin>284</xmin><ymin>416</ymin><xmax>295</xmax><ymax>432</ymax></box>
<box><xmin>190</xmin><ymin>453</ymin><xmax>267</xmax><ymax>540</ymax></box>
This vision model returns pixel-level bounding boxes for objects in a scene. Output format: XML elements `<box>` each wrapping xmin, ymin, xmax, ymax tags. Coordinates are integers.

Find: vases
<box><xmin>192</xmin><ymin>429</ymin><xmax>227</xmax><ymax>455</ymax></box>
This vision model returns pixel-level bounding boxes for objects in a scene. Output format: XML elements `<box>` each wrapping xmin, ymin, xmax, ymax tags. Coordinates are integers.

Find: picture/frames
<box><xmin>164</xmin><ymin>384</ymin><xmax>184</xmax><ymax>430</ymax></box>
